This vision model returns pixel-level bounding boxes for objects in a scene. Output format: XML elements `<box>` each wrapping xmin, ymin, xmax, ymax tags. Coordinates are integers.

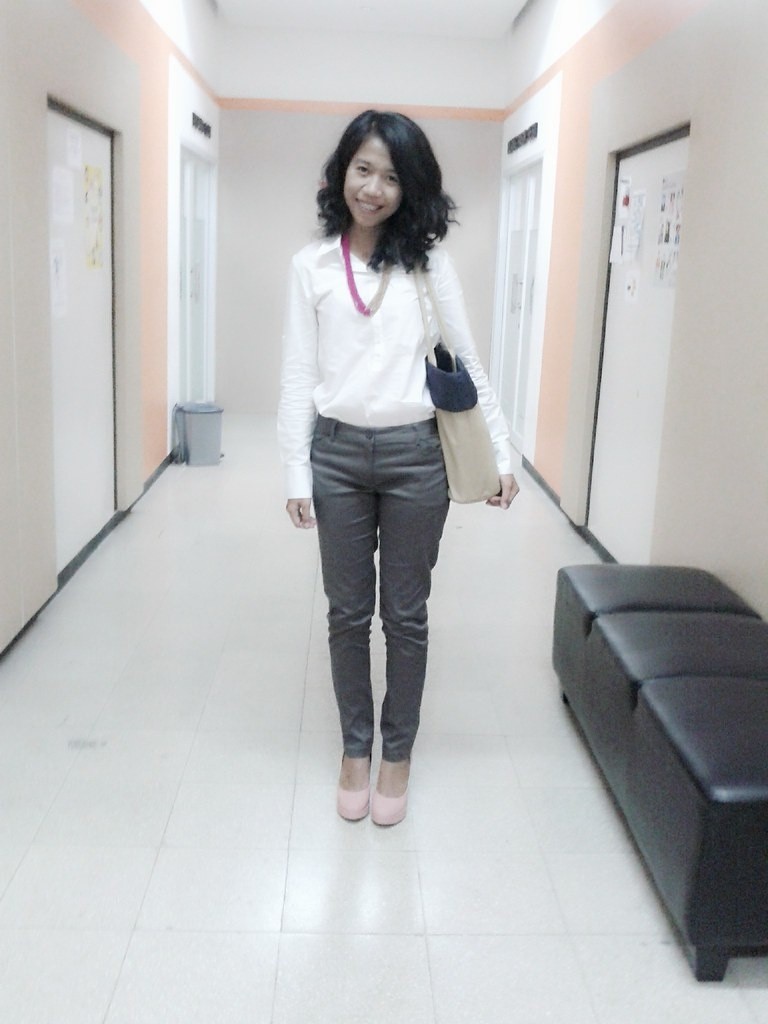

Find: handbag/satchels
<box><xmin>411</xmin><ymin>256</ymin><xmax>502</xmax><ymax>503</ymax></box>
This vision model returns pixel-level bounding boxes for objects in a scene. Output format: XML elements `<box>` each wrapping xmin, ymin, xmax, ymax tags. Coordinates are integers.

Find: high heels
<box><xmin>337</xmin><ymin>750</ymin><xmax>411</xmax><ymax>825</ymax></box>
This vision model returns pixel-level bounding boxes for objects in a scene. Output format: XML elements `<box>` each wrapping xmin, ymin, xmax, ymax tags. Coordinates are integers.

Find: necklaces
<box><xmin>340</xmin><ymin>226</ymin><xmax>393</xmax><ymax>317</ymax></box>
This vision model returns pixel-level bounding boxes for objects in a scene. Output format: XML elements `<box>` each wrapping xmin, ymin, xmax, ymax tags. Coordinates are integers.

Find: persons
<box><xmin>278</xmin><ymin>109</ymin><xmax>518</xmax><ymax>827</ymax></box>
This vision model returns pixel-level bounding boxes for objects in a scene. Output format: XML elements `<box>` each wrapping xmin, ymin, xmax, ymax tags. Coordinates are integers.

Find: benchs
<box><xmin>552</xmin><ymin>563</ymin><xmax>768</xmax><ymax>981</ymax></box>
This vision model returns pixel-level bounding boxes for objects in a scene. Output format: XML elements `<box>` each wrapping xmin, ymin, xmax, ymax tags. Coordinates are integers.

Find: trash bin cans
<box><xmin>175</xmin><ymin>403</ymin><xmax>224</xmax><ymax>466</ymax></box>
